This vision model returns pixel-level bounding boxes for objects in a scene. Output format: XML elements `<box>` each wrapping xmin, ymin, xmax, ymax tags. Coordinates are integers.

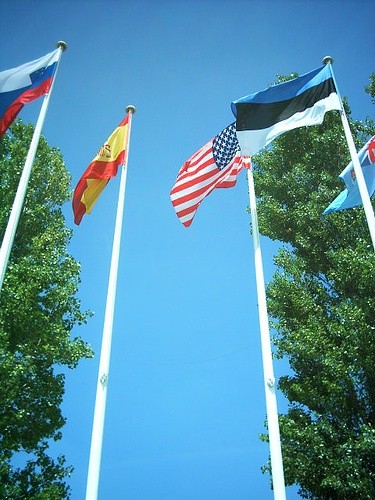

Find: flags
<box><xmin>321</xmin><ymin>132</ymin><xmax>375</xmax><ymax>216</ymax></box>
<box><xmin>168</xmin><ymin>120</ymin><xmax>253</xmax><ymax>227</ymax></box>
<box><xmin>71</xmin><ymin>109</ymin><xmax>131</xmax><ymax>226</ymax></box>
<box><xmin>0</xmin><ymin>44</ymin><xmax>63</xmax><ymax>141</ymax></box>
<box><xmin>229</xmin><ymin>60</ymin><xmax>343</xmax><ymax>157</ymax></box>
